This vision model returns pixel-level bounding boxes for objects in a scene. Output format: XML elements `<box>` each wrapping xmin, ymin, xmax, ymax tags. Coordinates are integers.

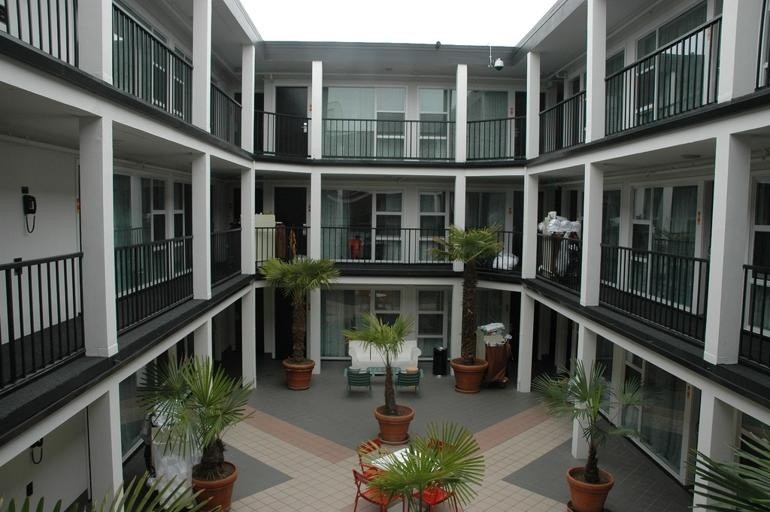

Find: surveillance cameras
<box><xmin>494</xmin><ymin>59</ymin><xmax>505</xmax><ymax>71</ymax></box>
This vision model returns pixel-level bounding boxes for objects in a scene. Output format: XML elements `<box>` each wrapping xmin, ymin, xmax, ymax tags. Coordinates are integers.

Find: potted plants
<box><xmin>137</xmin><ymin>344</ymin><xmax>254</xmax><ymax>510</ymax></box>
<box><xmin>345</xmin><ymin>307</ymin><xmax>431</xmax><ymax>445</ymax></box>
<box><xmin>258</xmin><ymin>255</ymin><xmax>341</xmax><ymax>392</ymax></box>
<box><xmin>522</xmin><ymin>360</ymin><xmax>664</xmax><ymax>512</ymax></box>
<box><xmin>423</xmin><ymin>216</ymin><xmax>508</xmax><ymax>395</ymax></box>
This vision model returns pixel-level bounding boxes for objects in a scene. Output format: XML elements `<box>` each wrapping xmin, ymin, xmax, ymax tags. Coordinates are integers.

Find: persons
<box><xmin>350</xmin><ymin>236</ymin><xmax>362</xmax><ymax>259</ymax></box>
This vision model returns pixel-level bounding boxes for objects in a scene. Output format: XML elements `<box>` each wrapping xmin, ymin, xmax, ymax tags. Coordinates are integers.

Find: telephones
<box><xmin>22</xmin><ymin>195</ymin><xmax>36</xmax><ymax>214</ymax></box>
<box><xmin>31</xmin><ymin>437</ymin><xmax>43</xmax><ymax>448</ymax></box>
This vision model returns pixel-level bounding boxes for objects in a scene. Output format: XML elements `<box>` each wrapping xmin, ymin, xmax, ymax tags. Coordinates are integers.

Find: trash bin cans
<box><xmin>432</xmin><ymin>346</ymin><xmax>448</xmax><ymax>376</ymax></box>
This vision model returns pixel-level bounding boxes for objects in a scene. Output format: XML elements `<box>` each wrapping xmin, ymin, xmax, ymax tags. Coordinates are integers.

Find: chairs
<box><xmin>343</xmin><ymin>360</ymin><xmax>424</xmax><ymax>395</ymax></box>
<box><xmin>347</xmin><ymin>433</ymin><xmax>463</xmax><ymax>512</ymax></box>
